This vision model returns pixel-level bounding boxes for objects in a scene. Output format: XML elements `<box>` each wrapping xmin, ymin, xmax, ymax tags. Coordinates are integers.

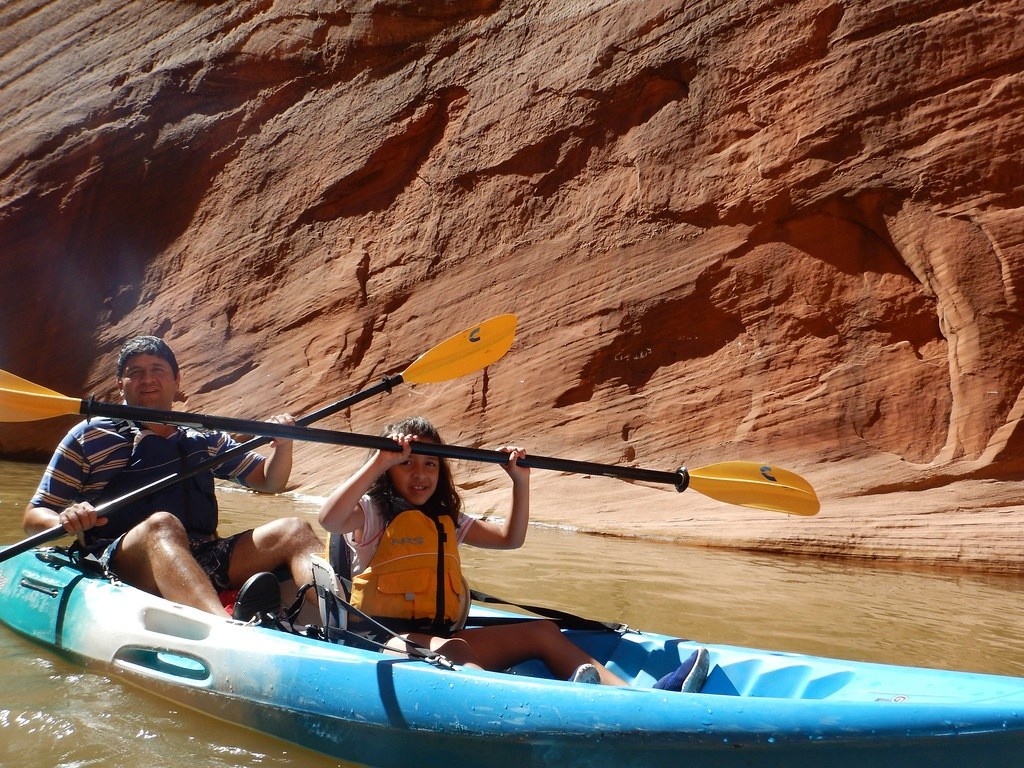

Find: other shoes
<box><xmin>663</xmin><ymin>648</ymin><xmax>710</xmax><ymax>693</ymax></box>
<box><xmin>565</xmin><ymin>663</ymin><xmax>600</xmax><ymax>685</ymax></box>
<box><xmin>232</xmin><ymin>571</ymin><xmax>280</xmax><ymax>631</ymax></box>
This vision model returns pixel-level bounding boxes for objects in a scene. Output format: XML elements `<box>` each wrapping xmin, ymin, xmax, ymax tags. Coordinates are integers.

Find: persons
<box><xmin>22</xmin><ymin>328</ymin><xmax>350</xmax><ymax>642</ymax></box>
<box><xmin>313</xmin><ymin>417</ymin><xmax>712</xmax><ymax>697</ymax></box>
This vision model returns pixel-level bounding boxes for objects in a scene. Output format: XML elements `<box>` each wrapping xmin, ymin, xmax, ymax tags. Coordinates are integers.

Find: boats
<box><xmin>0</xmin><ymin>535</ymin><xmax>1024</xmax><ymax>768</ymax></box>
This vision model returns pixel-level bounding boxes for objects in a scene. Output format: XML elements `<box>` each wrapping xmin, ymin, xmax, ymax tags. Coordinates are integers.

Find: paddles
<box><xmin>0</xmin><ymin>310</ymin><xmax>519</xmax><ymax>559</ymax></box>
<box><xmin>0</xmin><ymin>371</ymin><xmax>822</xmax><ymax>516</ymax></box>
